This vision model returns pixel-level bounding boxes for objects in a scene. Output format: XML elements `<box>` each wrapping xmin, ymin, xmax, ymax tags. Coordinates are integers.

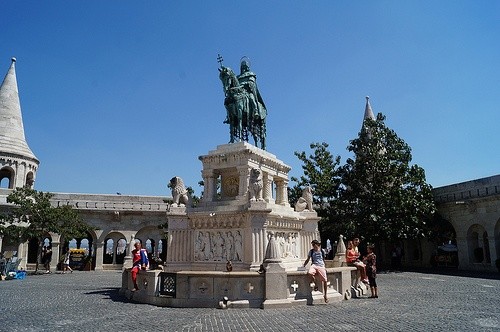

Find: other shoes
<box><xmin>368</xmin><ymin>296</ymin><xmax>379</xmax><ymax>298</ymax></box>
<box><xmin>131</xmin><ymin>287</ymin><xmax>139</xmax><ymax>292</ymax></box>
<box><xmin>362</xmin><ymin>280</ymin><xmax>368</xmax><ymax>284</ymax></box>
<box><xmin>325</xmin><ymin>296</ymin><xmax>330</xmax><ymax>303</ymax></box>
<box><xmin>141</xmin><ymin>266</ymin><xmax>146</xmax><ymax>271</ymax></box>
<box><xmin>315</xmin><ymin>286</ymin><xmax>318</xmax><ymax>291</ymax></box>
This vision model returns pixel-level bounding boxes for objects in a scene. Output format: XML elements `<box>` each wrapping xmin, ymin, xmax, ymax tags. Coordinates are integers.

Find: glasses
<box><xmin>312</xmin><ymin>244</ymin><xmax>316</xmax><ymax>245</ymax></box>
<box><xmin>355</xmin><ymin>241</ymin><xmax>360</xmax><ymax>243</ymax></box>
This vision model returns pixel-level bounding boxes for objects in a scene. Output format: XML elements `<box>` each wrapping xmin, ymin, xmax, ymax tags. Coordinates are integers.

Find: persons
<box><xmin>130</xmin><ymin>242</ymin><xmax>150</xmax><ymax>291</ymax></box>
<box><xmin>347</xmin><ymin>237</ymin><xmax>379</xmax><ymax>299</ymax></box>
<box><xmin>222</xmin><ymin>61</ymin><xmax>260</xmax><ymax>123</ymax></box>
<box><xmin>0</xmin><ymin>244</ymin><xmax>96</xmax><ymax>282</ymax></box>
<box><xmin>195</xmin><ymin>231</ymin><xmax>243</xmax><ymax>262</ymax></box>
<box><xmin>304</xmin><ymin>240</ymin><xmax>329</xmax><ymax>302</ymax></box>
<box><xmin>271</xmin><ymin>232</ymin><xmax>301</xmax><ymax>260</ymax></box>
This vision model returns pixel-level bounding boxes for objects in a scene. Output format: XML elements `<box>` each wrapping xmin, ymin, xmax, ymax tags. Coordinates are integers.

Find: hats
<box><xmin>311</xmin><ymin>240</ymin><xmax>322</xmax><ymax>245</ymax></box>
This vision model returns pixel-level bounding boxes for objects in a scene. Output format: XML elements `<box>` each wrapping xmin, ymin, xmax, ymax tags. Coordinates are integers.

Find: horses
<box><xmin>218</xmin><ymin>67</ymin><xmax>267</xmax><ymax>150</ymax></box>
